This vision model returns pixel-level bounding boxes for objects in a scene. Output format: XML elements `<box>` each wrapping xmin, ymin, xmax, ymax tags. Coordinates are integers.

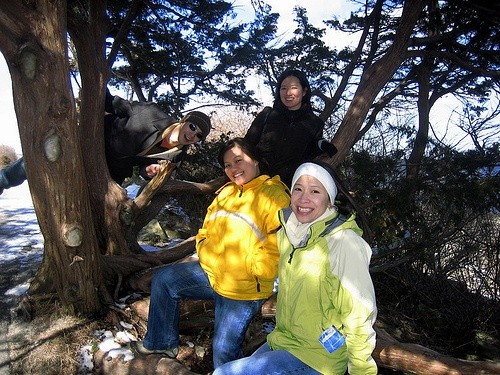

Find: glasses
<box><xmin>189</xmin><ymin>123</ymin><xmax>206</xmax><ymax>142</ymax></box>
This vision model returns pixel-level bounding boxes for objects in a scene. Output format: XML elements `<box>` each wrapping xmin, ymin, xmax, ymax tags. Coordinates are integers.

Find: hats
<box><xmin>179</xmin><ymin>111</ymin><xmax>211</xmax><ymax>138</ymax></box>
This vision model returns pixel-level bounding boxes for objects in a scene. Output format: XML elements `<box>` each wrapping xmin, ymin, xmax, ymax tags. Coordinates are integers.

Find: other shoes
<box><xmin>130</xmin><ymin>341</ymin><xmax>179</xmax><ymax>359</ymax></box>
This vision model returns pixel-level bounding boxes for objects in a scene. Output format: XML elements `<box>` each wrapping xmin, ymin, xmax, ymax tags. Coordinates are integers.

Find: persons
<box><xmin>130</xmin><ymin>138</ymin><xmax>292</xmax><ymax>372</ymax></box>
<box><xmin>0</xmin><ymin>88</ymin><xmax>211</xmax><ymax>193</ymax></box>
<box><xmin>240</xmin><ymin>69</ymin><xmax>337</xmax><ymax>188</ymax></box>
<box><xmin>213</xmin><ymin>162</ymin><xmax>378</xmax><ymax>375</ymax></box>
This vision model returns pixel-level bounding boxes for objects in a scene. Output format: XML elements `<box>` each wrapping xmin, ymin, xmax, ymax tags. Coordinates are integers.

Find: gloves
<box><xmin>320</xmin><ymin>140</ymin><xmax>338</xmax><ymax>158</ymax></box>
